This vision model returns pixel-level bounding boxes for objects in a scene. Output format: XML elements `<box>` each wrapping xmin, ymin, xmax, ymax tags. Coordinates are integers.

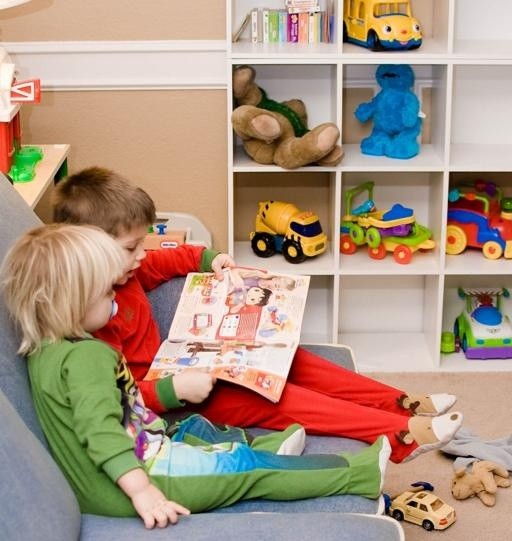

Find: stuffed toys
<box><xmin>355</xmin><ymin>64</ymin><xmax>424</xmax><ymax>159</ymax></box>
<box><xmin>451</xmin><ymin>458</ymin><xmax>511</xmax><ymax>507</ymax></box>
<box><xmin>232</xmin><ymin>66</ymin><xmax>346</xmax><ymax>171</ymax></box>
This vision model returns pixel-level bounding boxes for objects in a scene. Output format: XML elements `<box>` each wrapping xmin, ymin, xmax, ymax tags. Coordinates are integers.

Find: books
<box><xmin>144</xmin><ymin>270</ymin><xmax>310</xmax><ymax>406</ymax></box>
<box><xmin>232</xmin><ymin>1</ymin><xmax>334</xmax><ymax>43</ymax></box>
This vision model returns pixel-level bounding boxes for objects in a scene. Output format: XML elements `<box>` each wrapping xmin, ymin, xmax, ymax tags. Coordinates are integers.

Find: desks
<box><xmin>9</xmin><ymin>144</ymin><xmax>70</xmax><ymax>210</ymax></box>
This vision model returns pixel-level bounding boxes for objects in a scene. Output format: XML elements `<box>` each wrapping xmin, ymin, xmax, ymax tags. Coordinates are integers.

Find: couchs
<box><xmin>0</xmin><ymin>174</ymin><xmax>382</xmax><ymax>514</ymax></box>
<box><xmin>0</xmin><ymin>395</ymin><xmax>404</xmax><ymax>540</ymax></box>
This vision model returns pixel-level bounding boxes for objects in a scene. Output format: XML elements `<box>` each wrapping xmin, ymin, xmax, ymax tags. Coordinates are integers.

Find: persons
<box><xmin>49</xmin><ymin>166</ymin><xmax>462</xmax><ymax>465</ymax></box>
<box><xmin>0</xmin><ymin>224</ymin><xmax>391</xmax><ymax>530</ymax></box>
<box><xmin>226</xmin><ymin>339</ymin><xmax>287</xmax><ymax>352</ymax></box>
<box><xmin>223</xmin><ymin>284</ymin><xmax>272</xmax><ymax>315</ymax></box>
<box><xmin>218</xmin><ymin>268</ymin><xmax>296</xmax><ymax>294</ymax></box>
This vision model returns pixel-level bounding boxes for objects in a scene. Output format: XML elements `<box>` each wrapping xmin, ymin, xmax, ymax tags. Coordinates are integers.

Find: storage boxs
<box><xmin>0</xmin><ymin>103</ymin><xmax>23</xmax><ymax>173</ymax></box>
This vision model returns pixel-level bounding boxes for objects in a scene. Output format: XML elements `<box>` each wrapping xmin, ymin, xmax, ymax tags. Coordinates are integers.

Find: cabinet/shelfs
<box><xmin>228</xmin><ymin>2</ymin><xmax>512</xmax><ymax>373</ymax></box>
